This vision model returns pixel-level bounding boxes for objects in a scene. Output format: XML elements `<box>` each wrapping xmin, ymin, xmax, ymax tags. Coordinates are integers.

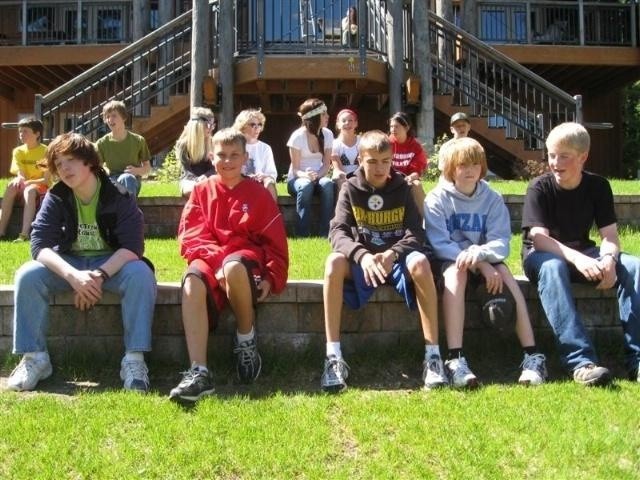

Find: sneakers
<box><xmin>442</xmin><ymin>356</ymin><xmax>478</xmax><ymax>391</ymax></box>
<box><xmin>421</xmin><ymin>354</ymin><xmax>448</xmax><ymax>391</ymax></box>
<box><xmin>566</xmin><ymin>361</ymin><xmax>611</xmax><ymax>389</ymax></box>
<box><xmin>169</xmin><ymin>366</ymin><xmax>217</xmax><ymax>403</ymax></box>
<box><xmin>7</xmin><ymin>350</ymin><xmax>55</xmax><ymax>393</ymax></box>
<box><xmin>234</xmin><ymin>333</ymin><xmax>263</xmax><ymax>385</ymax></box>
<box><xmin>320</xmin><ymin>352</ymin><xmax>350</xmax><ymax>396</ymax></box>
<box><xmin>119</xmin><ymin>356</ymin><xmax>153</xmax><ymax>391</ymax></box>
<box><xmin>515</xmin><ymin>352</ymin><xmax>549</xmax><ymax>385</ymax></box>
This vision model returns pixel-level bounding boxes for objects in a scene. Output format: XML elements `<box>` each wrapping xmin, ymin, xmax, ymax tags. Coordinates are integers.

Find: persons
<box><xmin>168</xmin><ymin>129</ymin><xmax>290</xmax><ymax>402</ymax></box>
<box><xmin>521</xmin><ymin>122</ymin><xmax>639</xmax><ymax>384</ymax></box>
<box><xmin>320</xmin><ymin>128</ymin><xmax>449</xmax><ymax>391</ymax></box>
<box><xmin>423</xmin><ymin>135</ymin><xmax>548</xmax><ymax>389</ymax></box>
<box><xmin>340</xmin><ymin>6</ymin><xmax>359</xmax><ymax>48</ymax></box>
<box><xmin>0</xmin><ymin>117</ymin><xmax>51</xmax><ymax>243</ymax></box>
<box><xmin>170</xmin><ymin>97</ymin><xmax>474</xmax><ymax>238</ymax></box>
<box><xmin>94</xmin><ymin>99</ymin><xmax>151</xmax><ymax>200</ymax></box>
<box><xmin>8</xmin><ymin>131</ymin><xmax>157</xmax><ymax>395</ymax></box>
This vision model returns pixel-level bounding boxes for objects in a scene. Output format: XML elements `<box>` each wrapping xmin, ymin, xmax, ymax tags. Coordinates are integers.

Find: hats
<box><xmin>476</xmin><ymin>280</ymin><xmax>518</xmax><ymax>342</ymax></box>
<box><xmin>449</xmin><ymin>112</ymin><xmax>470</xmax><ymax>127</ymax></box>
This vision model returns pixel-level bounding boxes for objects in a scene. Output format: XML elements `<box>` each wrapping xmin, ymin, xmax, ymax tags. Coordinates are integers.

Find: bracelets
<box><xmin>96</xmin><ymin>267</ymin><xmax>111</xmax><ymax>282</ymax></box>
<box><xmin>393</xmin><ymin>250</ymin><xmax>400</xmax><ymax>260</ymax></box>
<box><xmin>605</xmin><ymin>252</ymin><xmax>620</xmax><ymax>263</ymax></box>
